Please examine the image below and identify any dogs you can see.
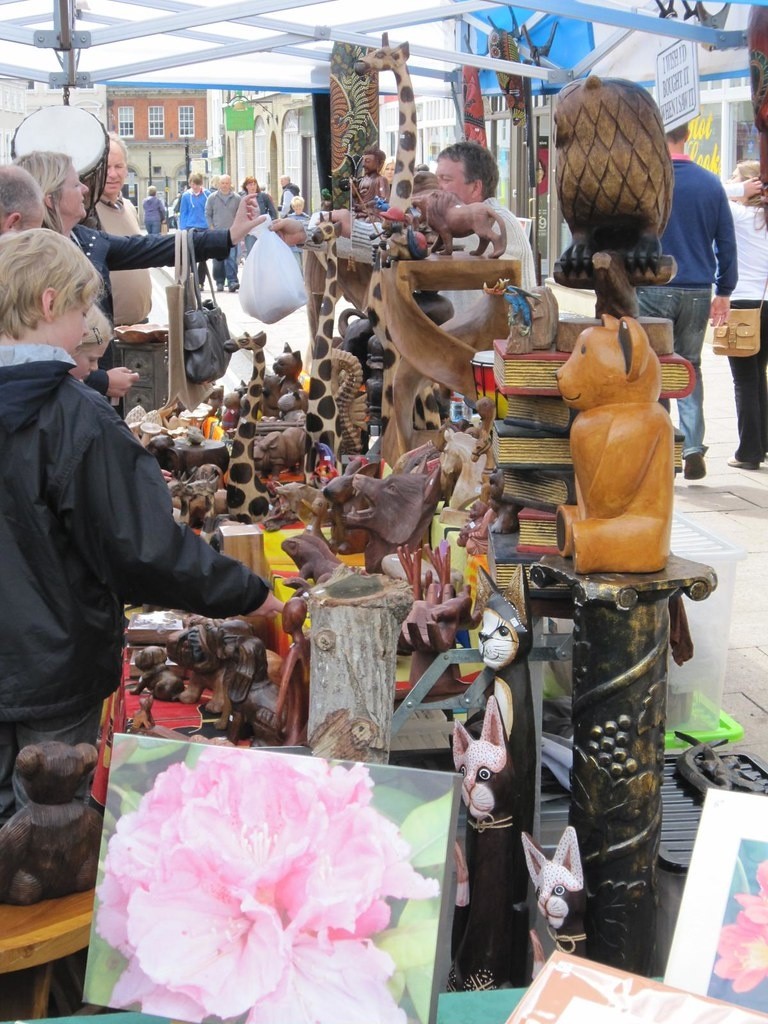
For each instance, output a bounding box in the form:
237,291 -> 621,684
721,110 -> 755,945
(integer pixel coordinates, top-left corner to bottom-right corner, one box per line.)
272,342 -> 303,395
128,645 -> 185,702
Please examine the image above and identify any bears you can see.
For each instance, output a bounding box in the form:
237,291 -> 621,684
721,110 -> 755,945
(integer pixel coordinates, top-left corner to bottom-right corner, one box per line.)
554,312 -> 676,574
0,738 -> 104,906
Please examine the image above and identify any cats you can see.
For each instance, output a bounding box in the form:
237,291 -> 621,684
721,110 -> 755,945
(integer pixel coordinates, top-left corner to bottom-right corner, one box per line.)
451,561 -> 594,988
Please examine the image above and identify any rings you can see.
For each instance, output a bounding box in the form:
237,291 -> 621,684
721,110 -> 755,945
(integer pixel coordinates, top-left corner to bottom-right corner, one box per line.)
755,182 -> 758,185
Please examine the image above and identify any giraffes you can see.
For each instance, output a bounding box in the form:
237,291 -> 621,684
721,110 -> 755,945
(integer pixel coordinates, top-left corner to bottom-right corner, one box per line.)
350,30 -> 442,471
297,211 -> 344,491
222,330 -> 272,523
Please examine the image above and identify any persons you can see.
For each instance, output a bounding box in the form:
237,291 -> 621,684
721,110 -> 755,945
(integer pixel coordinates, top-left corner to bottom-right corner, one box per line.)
355,148 -> 391,217
0,130 -> 311,827
638,119 -> 768,480
268,142 -> 537,336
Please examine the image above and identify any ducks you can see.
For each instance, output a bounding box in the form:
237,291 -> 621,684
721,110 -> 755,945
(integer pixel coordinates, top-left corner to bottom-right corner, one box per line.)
301,496 -> 332,552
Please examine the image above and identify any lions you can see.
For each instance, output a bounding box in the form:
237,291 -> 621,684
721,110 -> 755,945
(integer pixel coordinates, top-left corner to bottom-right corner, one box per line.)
411,191 -> 508,259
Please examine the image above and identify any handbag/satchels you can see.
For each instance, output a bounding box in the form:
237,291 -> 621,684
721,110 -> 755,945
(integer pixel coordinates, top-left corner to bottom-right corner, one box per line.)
239,214 -> 309,324
162,228 -> 232,414
712,307 -> 761,357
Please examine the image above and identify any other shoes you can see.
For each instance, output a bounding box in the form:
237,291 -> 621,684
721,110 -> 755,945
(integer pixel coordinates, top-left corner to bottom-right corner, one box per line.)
217,283 -> 224,291
728,459 -> 760,469
199,283 -> 204,291
684,453 -> 706,480
228,281 -> 240,292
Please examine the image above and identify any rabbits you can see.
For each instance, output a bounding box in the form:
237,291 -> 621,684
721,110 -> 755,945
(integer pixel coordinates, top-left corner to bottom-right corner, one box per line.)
322,459 -> 378,556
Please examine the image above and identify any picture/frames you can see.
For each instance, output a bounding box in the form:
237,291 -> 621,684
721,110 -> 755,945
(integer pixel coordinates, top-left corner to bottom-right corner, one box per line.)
663,785 -> 768,1014
